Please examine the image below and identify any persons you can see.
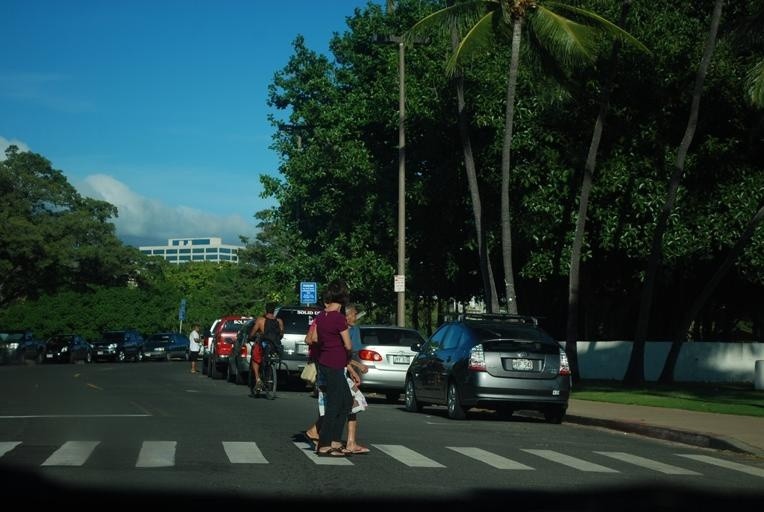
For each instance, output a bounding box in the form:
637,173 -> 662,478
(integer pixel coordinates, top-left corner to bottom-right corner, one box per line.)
346,304 -> 369,378
250,303 -> 284,390
311,280 -> 353,456
189,324 -> 205,374
305,291 -> 371,453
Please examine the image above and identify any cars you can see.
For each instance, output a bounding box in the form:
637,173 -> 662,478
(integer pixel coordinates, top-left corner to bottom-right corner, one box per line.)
225,319 -> 254,384
350,325 -> 426,401
141,333 -> 190,362
36,334 -> 94,364
203,315 -> 257,379
404,310 -> 571,424
0,330 -> 36,365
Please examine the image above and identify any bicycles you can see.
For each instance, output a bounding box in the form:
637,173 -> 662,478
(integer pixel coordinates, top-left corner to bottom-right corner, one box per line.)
248,337 -> 277,400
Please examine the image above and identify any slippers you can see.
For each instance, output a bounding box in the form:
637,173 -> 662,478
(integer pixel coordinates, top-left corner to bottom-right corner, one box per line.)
336,445 -> 353,456
346,444 -> 370,454
316,448 -> 346,458
304,432 -> 319,449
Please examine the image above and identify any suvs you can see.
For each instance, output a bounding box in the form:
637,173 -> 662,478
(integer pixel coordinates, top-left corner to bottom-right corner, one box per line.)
275,307 -> 329,388
89,331 -> 143,363
